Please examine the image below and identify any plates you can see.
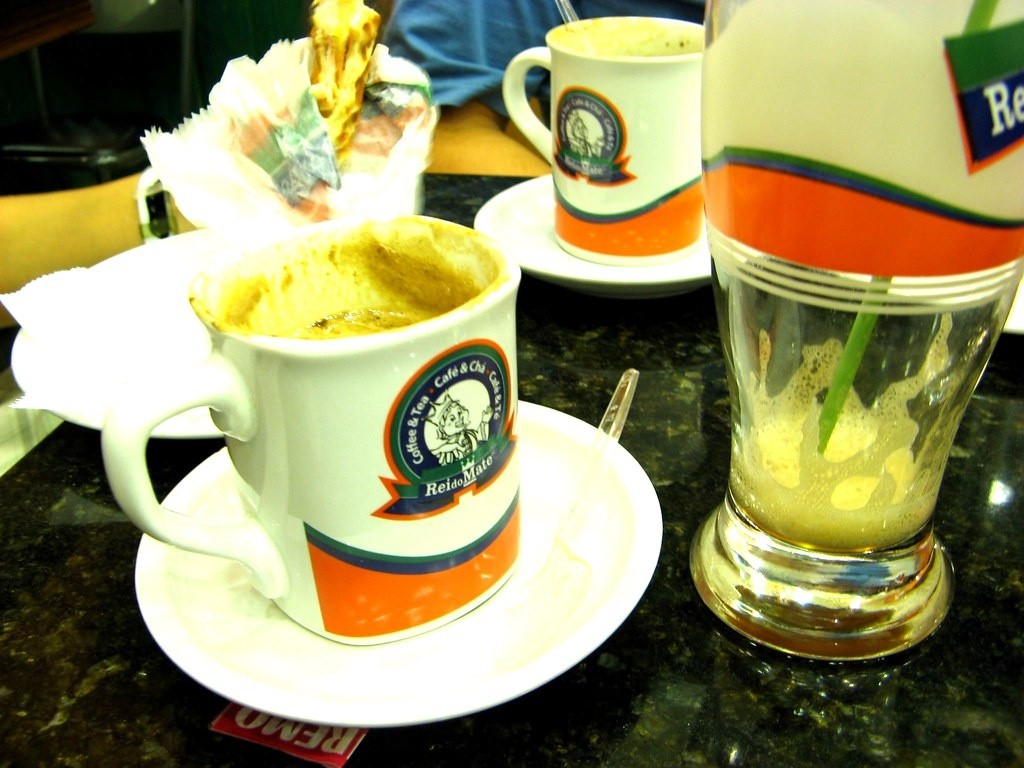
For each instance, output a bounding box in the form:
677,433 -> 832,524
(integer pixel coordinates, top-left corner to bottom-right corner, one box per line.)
133,398 -> 665,728
10,228 -> 297,440
474,174 -> 712,299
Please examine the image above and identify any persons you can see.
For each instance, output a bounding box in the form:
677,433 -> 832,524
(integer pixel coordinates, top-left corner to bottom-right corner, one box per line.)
0,162 -> 200,329
382,0 -> 706,177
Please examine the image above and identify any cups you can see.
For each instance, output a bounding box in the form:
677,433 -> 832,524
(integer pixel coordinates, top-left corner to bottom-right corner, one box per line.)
501,17 -> 705,266
101,214 -> 523,646
688,0 -> 1024,659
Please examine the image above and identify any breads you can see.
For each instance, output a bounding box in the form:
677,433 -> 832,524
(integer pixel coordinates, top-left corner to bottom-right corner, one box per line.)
307,0 -> 382,169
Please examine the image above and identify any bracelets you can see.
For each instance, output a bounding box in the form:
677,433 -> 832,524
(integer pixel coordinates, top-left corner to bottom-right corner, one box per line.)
136,165 -> 179,243
